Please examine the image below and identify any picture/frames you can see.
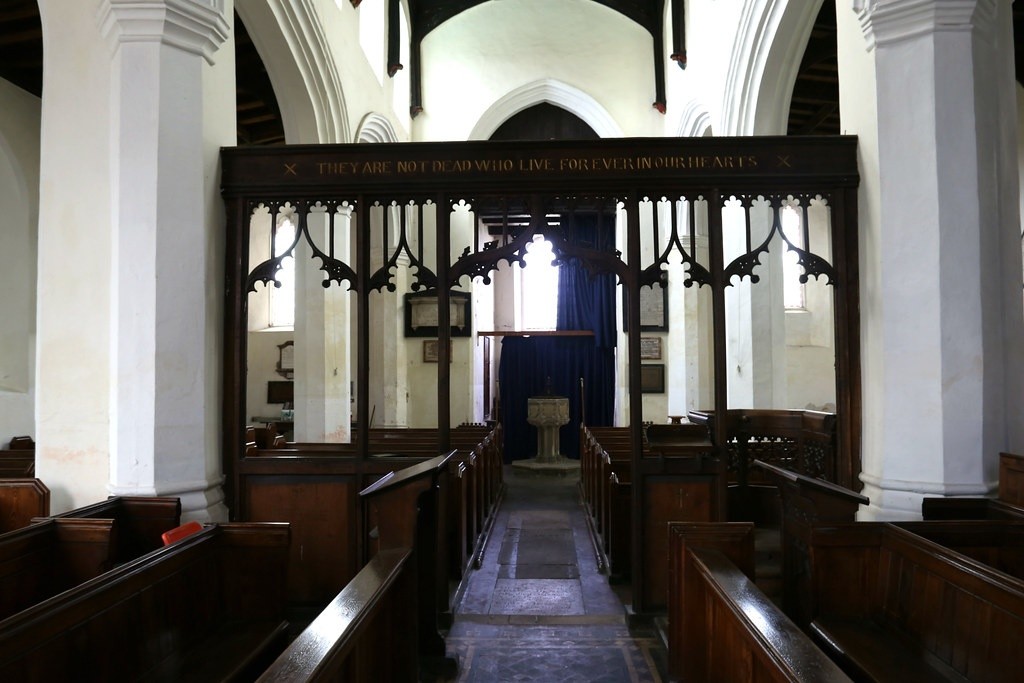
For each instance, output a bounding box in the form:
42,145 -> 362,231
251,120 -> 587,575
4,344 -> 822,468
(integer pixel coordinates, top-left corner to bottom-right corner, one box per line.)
642,364 -> 665,393
641,337 -> 661,360
423,340 -> 453,363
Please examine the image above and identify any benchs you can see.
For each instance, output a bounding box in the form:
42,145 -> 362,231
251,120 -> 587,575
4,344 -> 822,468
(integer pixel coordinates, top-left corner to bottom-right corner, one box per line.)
0,420 -> 504,683
579,409 -> 1024,683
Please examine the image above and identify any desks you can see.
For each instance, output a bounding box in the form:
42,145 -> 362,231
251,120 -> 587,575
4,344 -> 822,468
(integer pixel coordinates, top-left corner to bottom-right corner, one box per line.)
512,398 -> 581,470
